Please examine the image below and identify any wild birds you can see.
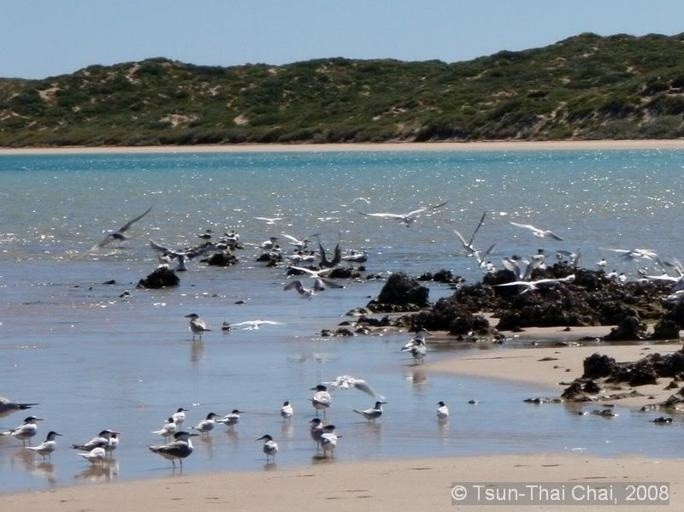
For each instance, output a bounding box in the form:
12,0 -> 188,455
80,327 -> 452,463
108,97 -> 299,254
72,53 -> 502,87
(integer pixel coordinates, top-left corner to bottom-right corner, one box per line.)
448,212 -> 684,296
148,407 -> 247,471
359,202 -> 448,229
184,313 -> 212,340
221,319 -> 282,330
308,375 -> 388,460
401,328 -> 433,363
281,399 -> 294,423
257,433 -> 278,462
149,217 -> 368,299
437,401 -> 449,421
352,197 -> 370,204
71,429 -> 121,465
77,207 -> 151,260
0,398 -> 64,461
